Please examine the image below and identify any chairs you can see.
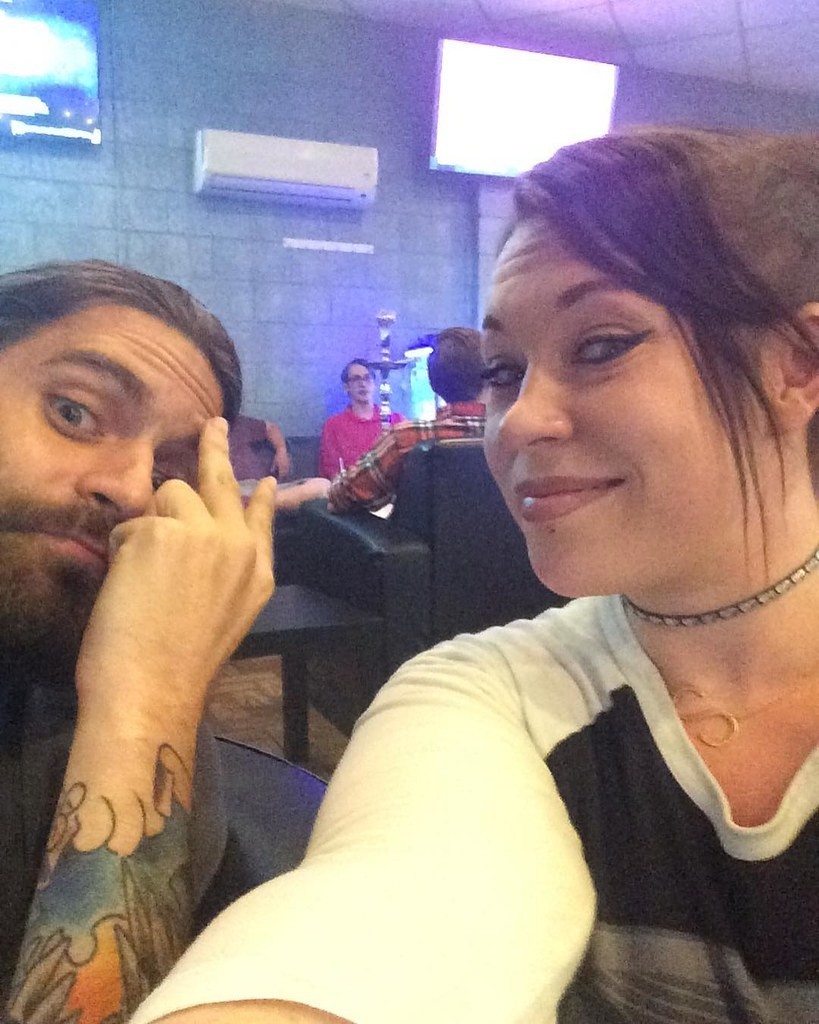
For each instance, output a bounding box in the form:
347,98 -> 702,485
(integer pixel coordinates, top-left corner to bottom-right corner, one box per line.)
190,735 -> 327,937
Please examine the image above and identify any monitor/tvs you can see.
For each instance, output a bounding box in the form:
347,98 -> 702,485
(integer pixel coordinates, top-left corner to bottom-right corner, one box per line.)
424,37 -> 621,182
0,0 -> 102,145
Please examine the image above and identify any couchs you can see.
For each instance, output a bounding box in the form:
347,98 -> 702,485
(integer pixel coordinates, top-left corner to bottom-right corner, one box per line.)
287,437 -> 576,741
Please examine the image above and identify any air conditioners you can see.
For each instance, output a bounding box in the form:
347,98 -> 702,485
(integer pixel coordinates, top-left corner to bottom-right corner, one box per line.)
191,128 -> 379,211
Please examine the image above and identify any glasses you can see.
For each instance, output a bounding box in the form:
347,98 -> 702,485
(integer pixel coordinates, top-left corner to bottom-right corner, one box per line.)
346,374 -> 373,382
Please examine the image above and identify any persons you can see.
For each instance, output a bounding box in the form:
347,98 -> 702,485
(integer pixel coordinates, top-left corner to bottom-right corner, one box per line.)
127,125 -> 819,1024
0,261 -> 279,1021
228,325 -> 494,586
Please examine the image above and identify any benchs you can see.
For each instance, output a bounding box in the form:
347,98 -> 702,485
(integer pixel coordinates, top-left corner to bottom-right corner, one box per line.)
273,435 -> 321,583
230,585 -> 372,766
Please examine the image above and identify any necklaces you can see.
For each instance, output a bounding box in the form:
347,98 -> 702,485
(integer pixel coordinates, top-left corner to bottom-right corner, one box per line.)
621,544 -> 819,629
666,684 -> 802,748
